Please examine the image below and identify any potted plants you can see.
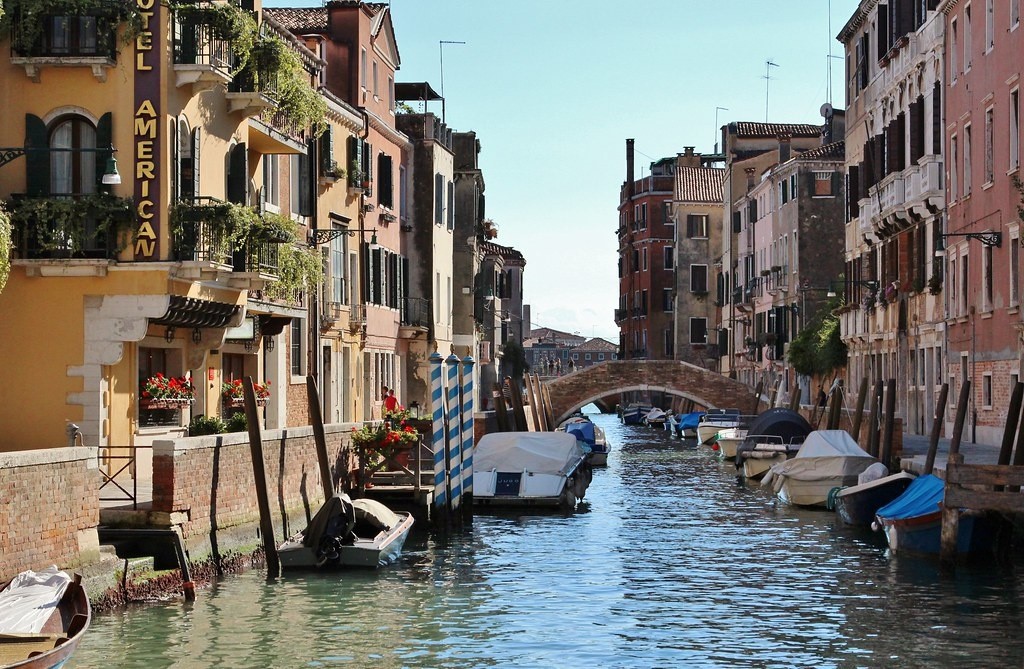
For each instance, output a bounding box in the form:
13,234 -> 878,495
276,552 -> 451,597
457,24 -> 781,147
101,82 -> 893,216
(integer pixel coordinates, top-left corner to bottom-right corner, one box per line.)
758,332 -> 777,347
771,266 -> 781,272
1,188 -> 301,269
165,0 -> 332,142
831,309 -> 843,316
928,275 -> 943,297
324,159 -> 370,188
417,414 -> 433,432
840,306 -> 851,313
848,301 -> 860,310
473,217 -> 499,241
760,270 -> 770,276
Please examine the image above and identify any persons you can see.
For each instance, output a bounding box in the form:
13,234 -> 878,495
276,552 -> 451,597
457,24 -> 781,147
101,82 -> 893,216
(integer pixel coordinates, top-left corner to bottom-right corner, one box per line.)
567,357 -> 575,374
383,386 -> 389,400
384,389 -> 399,413
818,385 -> 827,406
538,357 -> 561,376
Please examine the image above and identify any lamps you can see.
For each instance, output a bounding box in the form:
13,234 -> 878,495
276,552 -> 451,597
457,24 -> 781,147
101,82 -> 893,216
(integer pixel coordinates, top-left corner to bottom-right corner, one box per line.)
410,401 -> 420,420
484,309 -> 512,322
703,319 -> 751,338
0,142 -> 122,185
934,230 -> 1003,257
487,326 -> 514,337
769,304 -> 800,318
309,227 -> 379,250
472,284 -> 494,300
165,324 -> 177,343
826,280 -> 880,298
192,327 -> 202,346
244,341 -> 252,352
267,335 -> 274,353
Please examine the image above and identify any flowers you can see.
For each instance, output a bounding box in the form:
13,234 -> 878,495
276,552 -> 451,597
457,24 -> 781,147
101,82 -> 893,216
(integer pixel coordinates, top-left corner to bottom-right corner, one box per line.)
221,376 -> 272,400
347,404 -> 419,489
885,286 -> 898,305
137,372 -> 198,400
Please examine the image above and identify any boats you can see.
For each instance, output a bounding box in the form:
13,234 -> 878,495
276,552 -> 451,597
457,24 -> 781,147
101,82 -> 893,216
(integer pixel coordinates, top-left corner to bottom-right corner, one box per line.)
829,470 -> 917,526
278,498 -> 415,570
470,432 -> 594,507
760,430 -> 881,507
874,475 -> 974,556
554,413 -> 611,467
736,407 -> 813,480
0,565 -> 92,669
623,403 -> 707,438
696,408 -> 749,459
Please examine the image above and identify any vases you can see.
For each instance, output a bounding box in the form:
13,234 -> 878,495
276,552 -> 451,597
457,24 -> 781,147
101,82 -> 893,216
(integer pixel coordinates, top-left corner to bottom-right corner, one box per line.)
225,397 -> 271,407
877,58 -> 890,69
156,400 -> 167,408
166,400 -> 178,409
139,399 -> 157,409
386,441 -> 413,471
352,469 -> 373,489
888,47 -> 900,59
176,400 -> 188,410
895,37 -> 909,48
